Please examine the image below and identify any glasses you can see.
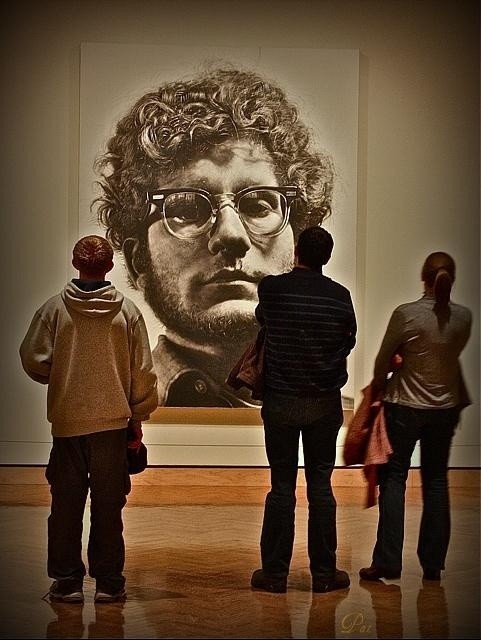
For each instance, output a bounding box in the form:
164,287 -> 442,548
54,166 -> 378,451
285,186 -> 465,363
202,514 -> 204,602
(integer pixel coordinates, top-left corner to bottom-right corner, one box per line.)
141,183 -> 297,240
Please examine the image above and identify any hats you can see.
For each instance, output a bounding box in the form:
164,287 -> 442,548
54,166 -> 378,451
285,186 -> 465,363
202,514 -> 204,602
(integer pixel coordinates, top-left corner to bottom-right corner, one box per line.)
127,435 -> 146,474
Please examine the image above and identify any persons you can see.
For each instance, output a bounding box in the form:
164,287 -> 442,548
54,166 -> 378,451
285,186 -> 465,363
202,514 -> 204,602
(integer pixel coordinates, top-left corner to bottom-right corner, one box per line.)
357,248 -> 471,584
43,602 -> 129,640
20,236 -> 158,603
250,226 -> 352,593
87,60 -> 354,409
254,588 -> 352,639
358,578 -> 453,640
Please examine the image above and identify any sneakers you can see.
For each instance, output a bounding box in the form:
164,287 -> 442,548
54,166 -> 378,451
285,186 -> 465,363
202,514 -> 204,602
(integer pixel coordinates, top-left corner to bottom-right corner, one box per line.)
250,569 -> 289,594
93,578 -> 127,603
311,570 -> 349,593
42,579 -> 84,604
359,565 -> 401,580
422,569 -> 441,581
